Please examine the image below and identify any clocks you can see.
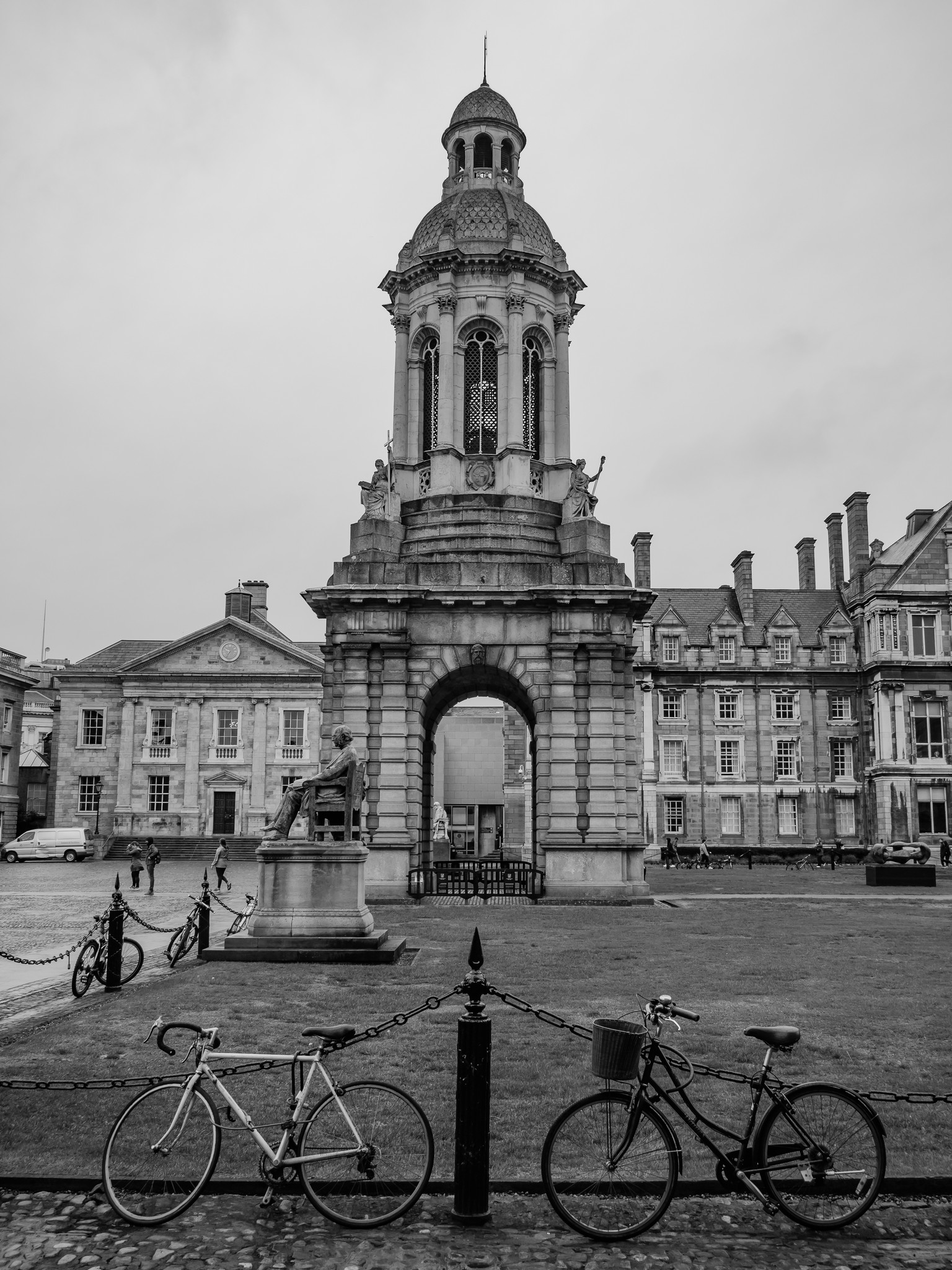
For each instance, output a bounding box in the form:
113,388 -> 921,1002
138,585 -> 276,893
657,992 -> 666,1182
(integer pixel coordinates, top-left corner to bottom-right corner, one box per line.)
219,641 -> 241,662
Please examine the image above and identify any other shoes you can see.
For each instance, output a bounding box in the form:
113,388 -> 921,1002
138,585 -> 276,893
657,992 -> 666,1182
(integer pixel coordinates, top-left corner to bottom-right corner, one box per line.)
834,861 -> 837,865
944,866 -> 948,869
697,866 -> 703,869
144,891 -> 154,895
709,866 -> 713,869
129,885 -> 140,890
227,882 -> 231,890
823,863 -> 826,866
663,864 -> 670,867
839,864 -> 844,867
816,866 -> 821,867
212,887 -> 220,892
948,863 -> 952,867
939,866 -> 945,868
670,865 -> 675,867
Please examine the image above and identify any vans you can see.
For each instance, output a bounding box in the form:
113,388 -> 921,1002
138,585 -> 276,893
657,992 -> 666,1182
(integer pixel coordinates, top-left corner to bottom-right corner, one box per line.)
1,826 -> 95,863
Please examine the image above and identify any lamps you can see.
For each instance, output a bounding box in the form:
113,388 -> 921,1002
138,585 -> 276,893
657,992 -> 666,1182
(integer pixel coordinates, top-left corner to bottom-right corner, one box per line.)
934,751 -> 939,757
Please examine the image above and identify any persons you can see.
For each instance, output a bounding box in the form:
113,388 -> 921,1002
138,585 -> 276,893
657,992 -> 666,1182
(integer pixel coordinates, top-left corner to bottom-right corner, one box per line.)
260,725 -> 357,841
432,802 -> 447,833
663,837 -> 679,867
697,839 -> 713,869
126,841 -> 145,890
567,459 -> 603,518
939,838 -> 952,869
357,446 -> 396,521
144,836 -> 158,895
497,824 -> 503,849
211,838 -> 232,892
834,839 -> 845,867
815,838 -> 826,868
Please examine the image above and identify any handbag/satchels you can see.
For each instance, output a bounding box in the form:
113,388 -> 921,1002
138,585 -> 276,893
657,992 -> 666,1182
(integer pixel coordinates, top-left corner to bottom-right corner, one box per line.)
815,848 -> 821,853
150,844 -> 161,864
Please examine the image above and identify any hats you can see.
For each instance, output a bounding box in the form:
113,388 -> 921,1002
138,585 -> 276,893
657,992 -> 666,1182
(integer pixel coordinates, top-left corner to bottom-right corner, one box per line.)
818,838 -> 822,841
837,839 -> 842,843
145,836 -> 153,846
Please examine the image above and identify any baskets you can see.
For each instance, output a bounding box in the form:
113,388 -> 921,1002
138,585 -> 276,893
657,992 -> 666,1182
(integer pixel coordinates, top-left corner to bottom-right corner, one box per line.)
591,1019 -> 649,1081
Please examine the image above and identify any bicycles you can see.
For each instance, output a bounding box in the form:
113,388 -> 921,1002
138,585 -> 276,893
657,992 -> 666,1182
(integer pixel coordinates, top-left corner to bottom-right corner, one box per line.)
707,854 -> 734,870
101,1014 -> 434,1230
225,892 -> 258,937
675,853 -> 708,870
786,854 -> 814,871
162,894 -> 215,969
539,992 -> 888,1244
70,915 -> 144,998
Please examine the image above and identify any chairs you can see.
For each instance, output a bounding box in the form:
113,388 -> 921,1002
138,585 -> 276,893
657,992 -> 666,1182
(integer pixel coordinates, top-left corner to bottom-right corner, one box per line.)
306,761 -> 367,841
